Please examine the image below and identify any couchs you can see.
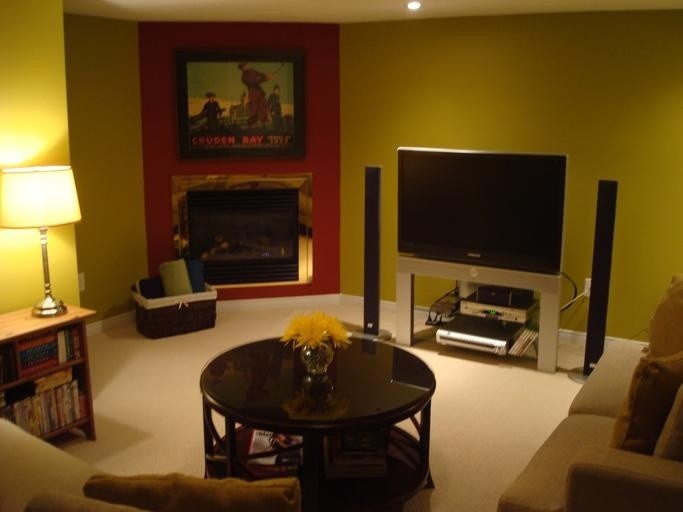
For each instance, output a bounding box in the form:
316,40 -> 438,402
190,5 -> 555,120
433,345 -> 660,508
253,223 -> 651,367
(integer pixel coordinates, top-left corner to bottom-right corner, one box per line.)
0,417 -> 151,511
497,338 -> 683,512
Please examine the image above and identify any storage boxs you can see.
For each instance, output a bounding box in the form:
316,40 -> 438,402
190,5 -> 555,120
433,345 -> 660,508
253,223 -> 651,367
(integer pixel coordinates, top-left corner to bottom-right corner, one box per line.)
133,282 -> 218,339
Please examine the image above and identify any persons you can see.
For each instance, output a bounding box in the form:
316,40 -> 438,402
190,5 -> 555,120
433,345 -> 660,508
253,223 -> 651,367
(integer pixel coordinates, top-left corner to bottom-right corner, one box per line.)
236,61 -> 273,126
266,83 -> 284,129
195,91 -> 224,130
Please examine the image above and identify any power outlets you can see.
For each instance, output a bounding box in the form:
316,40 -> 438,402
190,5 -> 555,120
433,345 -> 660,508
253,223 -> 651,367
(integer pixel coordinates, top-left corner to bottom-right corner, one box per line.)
584,279 -> 591,297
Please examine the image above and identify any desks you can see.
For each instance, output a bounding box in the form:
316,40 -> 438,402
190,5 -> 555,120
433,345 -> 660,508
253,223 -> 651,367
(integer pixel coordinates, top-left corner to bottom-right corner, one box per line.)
395,256 -> 561,373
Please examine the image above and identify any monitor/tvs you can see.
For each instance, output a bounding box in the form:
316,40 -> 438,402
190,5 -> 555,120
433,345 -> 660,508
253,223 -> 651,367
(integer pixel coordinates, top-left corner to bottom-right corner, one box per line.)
397,147 -> 568,274
176,50 -> 306,160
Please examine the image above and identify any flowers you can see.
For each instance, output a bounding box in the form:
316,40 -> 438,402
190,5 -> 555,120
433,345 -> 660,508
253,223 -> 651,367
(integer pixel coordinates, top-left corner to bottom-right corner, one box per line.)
281,391 -> 347,420
280,312 -> 352,349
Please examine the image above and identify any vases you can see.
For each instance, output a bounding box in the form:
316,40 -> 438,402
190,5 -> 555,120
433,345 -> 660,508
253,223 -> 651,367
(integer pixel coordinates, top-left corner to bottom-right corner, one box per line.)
301,342 -> 334,375
303,375 -> 334,397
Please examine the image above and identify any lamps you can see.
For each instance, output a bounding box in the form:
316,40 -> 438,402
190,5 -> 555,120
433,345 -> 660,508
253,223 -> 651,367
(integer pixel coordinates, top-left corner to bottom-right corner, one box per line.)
0,166 -> 82,318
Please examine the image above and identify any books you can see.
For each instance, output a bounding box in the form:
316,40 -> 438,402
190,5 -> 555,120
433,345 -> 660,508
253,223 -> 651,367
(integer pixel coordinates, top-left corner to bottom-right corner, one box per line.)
247,428 -> 306,471
0,325 -> 89,440
337,429 -> 376,456
506,317 -> 541,358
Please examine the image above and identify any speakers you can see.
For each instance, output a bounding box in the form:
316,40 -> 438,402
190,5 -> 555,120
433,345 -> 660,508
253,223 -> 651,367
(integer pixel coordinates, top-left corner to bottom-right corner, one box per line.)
354,166 -> 392,341
568,180 -> 618,385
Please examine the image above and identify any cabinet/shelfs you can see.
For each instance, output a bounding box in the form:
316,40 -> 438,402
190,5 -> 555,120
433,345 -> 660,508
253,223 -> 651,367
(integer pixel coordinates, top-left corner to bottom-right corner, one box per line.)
0,305 -> 98,441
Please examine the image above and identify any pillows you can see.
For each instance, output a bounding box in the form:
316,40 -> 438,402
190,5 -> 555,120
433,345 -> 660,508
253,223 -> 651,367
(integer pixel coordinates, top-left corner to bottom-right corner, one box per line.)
609,349 -> 683,455
645,273 -> 683,357
82,472 -> 302,512
